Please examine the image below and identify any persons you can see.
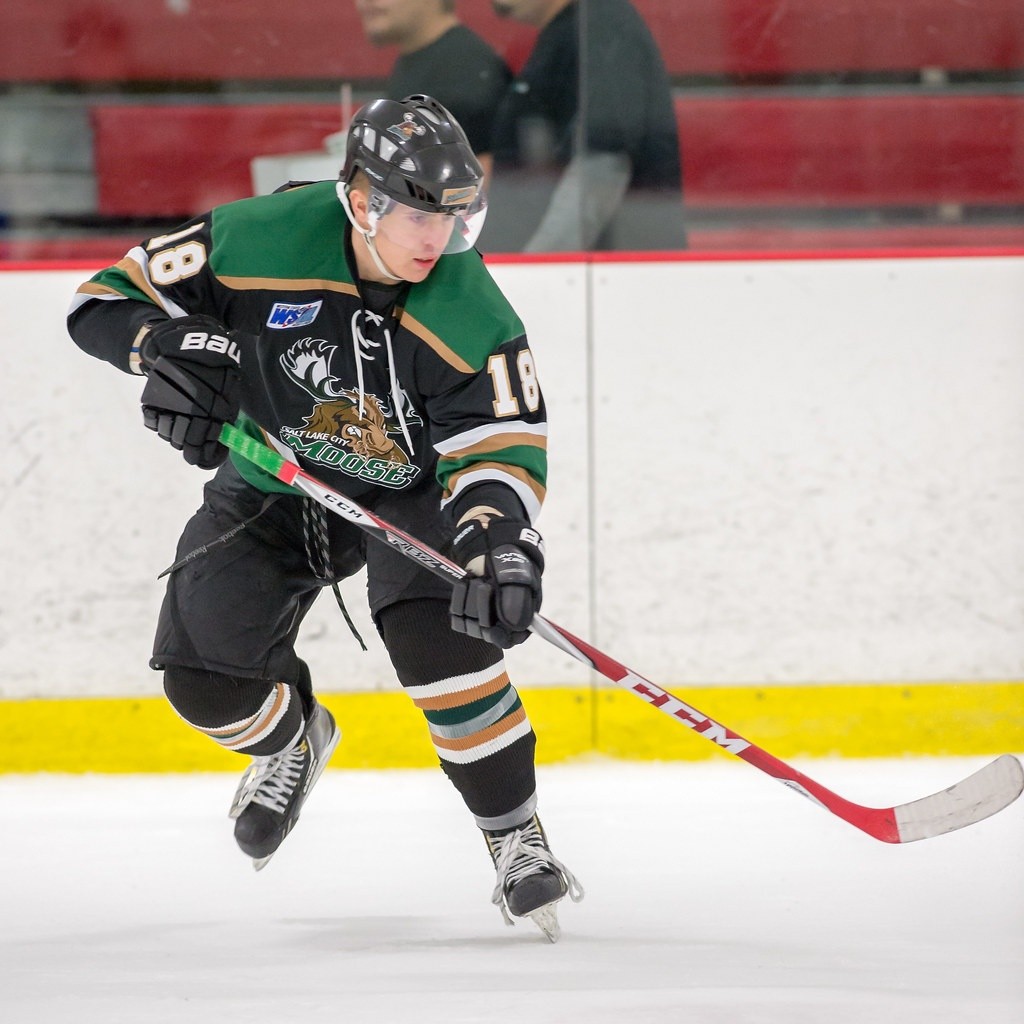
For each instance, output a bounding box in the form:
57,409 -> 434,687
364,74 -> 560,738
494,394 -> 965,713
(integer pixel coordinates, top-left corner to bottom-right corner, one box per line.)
66,94 -> 586,943
466,0 -> 688,256
356,0 -> 515,192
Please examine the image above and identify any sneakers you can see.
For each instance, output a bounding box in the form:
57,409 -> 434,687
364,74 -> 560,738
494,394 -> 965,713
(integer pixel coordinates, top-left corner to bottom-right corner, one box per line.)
228,657 -> 340,872
481,810 -> 585,943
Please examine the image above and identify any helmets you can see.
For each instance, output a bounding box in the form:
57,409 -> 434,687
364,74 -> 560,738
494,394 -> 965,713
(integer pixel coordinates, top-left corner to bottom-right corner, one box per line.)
344,94 -> 485,213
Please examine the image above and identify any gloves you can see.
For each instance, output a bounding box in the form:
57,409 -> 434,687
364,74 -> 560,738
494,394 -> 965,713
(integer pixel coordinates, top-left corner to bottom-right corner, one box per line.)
141,314 -> 243,470
439,518 -> 546,649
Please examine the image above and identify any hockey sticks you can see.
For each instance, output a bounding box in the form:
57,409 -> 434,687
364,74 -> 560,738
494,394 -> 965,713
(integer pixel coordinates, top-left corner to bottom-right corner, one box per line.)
223,405 -> 1024,845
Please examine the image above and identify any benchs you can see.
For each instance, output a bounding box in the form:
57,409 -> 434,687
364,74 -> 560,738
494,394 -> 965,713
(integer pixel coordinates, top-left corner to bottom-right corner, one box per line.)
0,0 -> 1024,246
